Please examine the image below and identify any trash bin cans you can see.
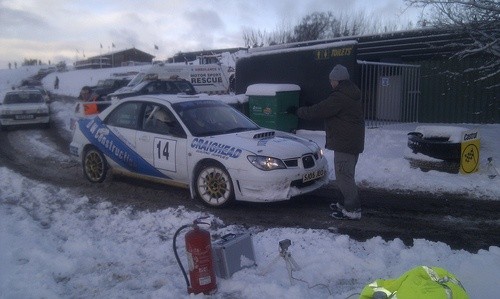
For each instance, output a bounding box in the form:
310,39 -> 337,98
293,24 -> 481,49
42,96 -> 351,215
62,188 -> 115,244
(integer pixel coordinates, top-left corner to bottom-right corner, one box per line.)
244,85 -> 300,135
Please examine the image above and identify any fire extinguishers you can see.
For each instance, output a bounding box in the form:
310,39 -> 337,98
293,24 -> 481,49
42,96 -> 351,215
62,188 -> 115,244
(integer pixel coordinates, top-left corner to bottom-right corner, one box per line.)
172,215 -> 218,295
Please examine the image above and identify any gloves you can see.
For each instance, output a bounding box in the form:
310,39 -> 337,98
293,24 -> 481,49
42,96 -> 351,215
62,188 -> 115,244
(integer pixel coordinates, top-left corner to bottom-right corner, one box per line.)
286,105 -> 297,116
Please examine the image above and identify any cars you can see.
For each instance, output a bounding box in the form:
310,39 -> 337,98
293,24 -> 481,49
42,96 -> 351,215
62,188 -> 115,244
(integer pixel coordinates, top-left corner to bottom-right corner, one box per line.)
0,90 -> 50,128
69,94 -> 331,209
99,76 -> 197,113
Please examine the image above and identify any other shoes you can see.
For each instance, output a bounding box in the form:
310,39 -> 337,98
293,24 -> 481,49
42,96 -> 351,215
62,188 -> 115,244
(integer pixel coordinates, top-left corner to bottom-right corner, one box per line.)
331,211 -> 351,219
329,203 -> 342,212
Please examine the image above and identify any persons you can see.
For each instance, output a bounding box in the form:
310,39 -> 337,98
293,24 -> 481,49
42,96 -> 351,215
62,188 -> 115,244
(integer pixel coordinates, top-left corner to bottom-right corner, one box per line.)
80,86 -> 109,115
287,65 -> 366,220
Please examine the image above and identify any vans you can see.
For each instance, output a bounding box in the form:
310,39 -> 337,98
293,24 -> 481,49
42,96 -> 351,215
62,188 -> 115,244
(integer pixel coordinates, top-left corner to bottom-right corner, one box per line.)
127,63 -> 231,96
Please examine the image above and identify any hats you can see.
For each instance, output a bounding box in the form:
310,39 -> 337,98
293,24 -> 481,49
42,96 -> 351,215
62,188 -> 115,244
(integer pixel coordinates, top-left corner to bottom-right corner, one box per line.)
328,64 -> 350,80
